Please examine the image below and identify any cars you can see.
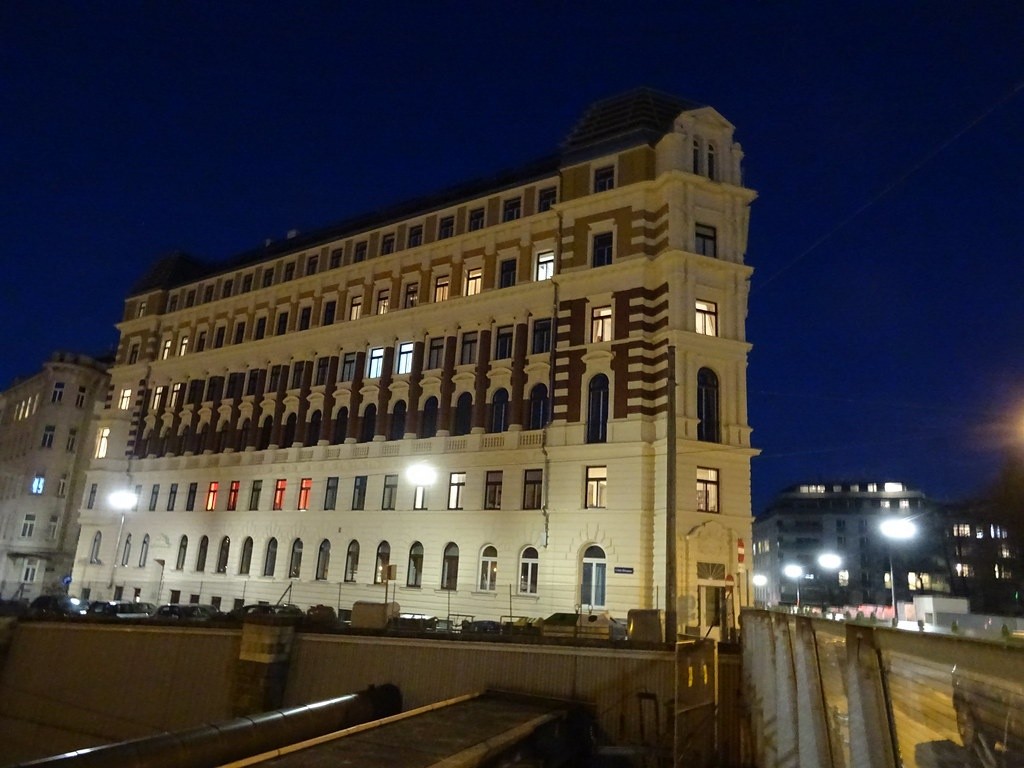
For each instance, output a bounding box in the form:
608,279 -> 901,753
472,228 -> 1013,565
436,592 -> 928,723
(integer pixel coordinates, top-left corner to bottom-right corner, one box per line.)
26,594 -> 90,617
225,604 -> 303,615
85,600 -> 158,618
153,604 -> 219,621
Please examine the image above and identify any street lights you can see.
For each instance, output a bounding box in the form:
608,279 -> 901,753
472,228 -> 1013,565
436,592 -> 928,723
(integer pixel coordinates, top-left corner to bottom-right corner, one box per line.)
789,568 -> 799,605
885,523 -> 904,617
